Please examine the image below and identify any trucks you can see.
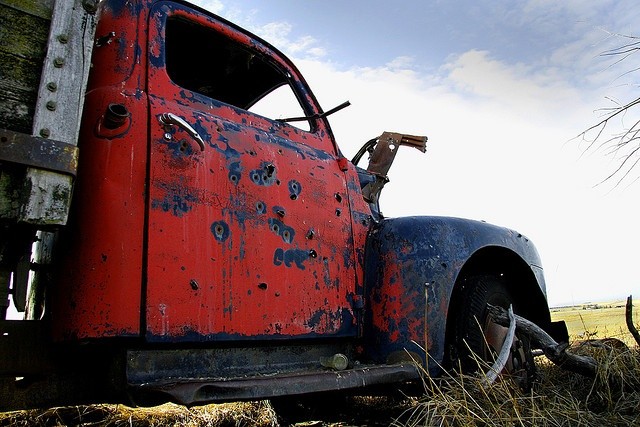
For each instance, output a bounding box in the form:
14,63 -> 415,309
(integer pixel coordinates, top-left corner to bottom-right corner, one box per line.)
2,0 -> 573,424
